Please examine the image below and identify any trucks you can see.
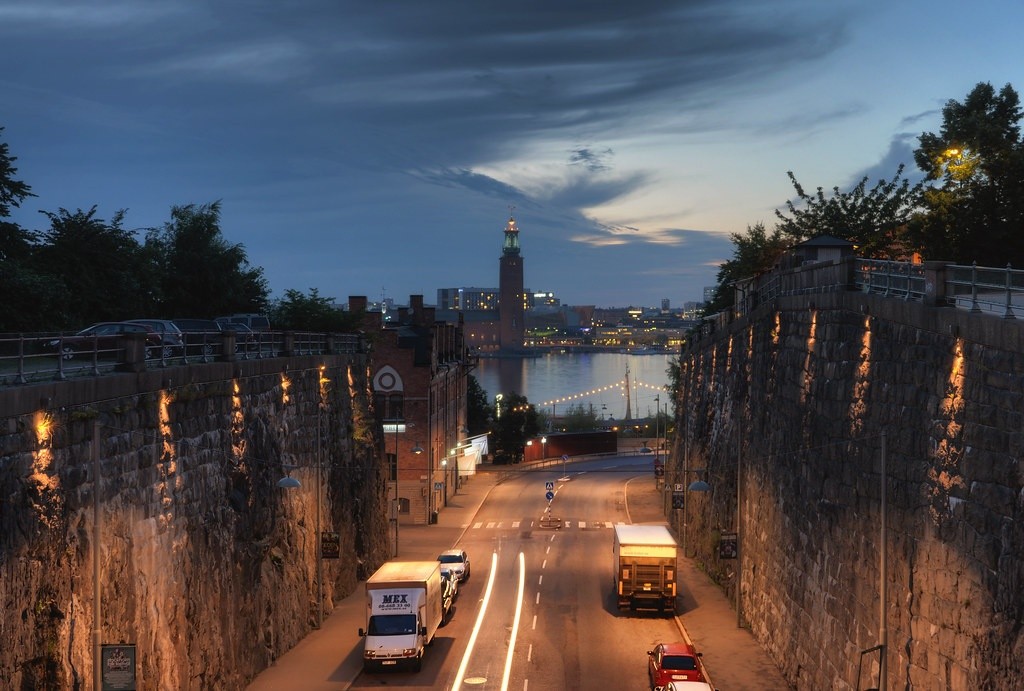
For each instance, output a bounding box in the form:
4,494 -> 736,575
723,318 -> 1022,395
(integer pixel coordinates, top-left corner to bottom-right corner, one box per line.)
611,522 -> 679,617
356,561 -> 444,672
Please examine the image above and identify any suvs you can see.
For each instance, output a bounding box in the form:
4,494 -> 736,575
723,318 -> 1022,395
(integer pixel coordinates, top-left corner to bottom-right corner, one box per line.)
436,549 -> 471,583
100,318 -> 184,358
173,313 -> 272,356
41,322 -> 162,361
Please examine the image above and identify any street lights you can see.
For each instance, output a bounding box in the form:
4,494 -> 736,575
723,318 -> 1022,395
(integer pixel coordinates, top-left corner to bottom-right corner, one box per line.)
541,436 -> 547,469
653,394 -> 659,463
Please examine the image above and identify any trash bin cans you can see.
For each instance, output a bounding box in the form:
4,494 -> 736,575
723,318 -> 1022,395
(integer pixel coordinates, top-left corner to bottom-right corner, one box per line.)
431,510 -> 440,524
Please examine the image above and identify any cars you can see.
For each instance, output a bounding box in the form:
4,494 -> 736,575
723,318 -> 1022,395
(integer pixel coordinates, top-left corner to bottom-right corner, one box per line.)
440,567 -> 459,596
647,642 -> 704,691
440,575 -> 454,627
662,682 -> 720,691
